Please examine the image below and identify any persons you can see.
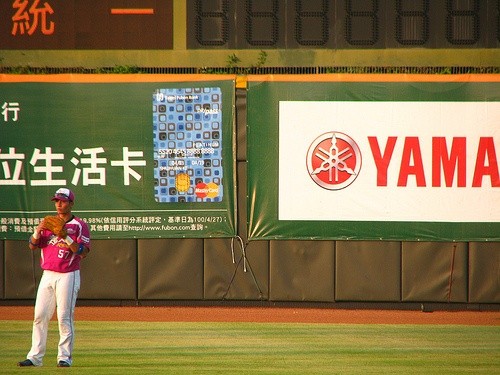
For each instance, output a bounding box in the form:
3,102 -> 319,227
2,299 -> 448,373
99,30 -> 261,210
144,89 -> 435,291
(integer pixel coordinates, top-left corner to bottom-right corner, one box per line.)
16,187 -> 91,368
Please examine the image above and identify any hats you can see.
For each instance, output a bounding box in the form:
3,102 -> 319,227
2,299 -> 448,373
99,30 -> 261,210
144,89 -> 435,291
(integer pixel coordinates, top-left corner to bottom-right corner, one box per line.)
51,188 -> 74,201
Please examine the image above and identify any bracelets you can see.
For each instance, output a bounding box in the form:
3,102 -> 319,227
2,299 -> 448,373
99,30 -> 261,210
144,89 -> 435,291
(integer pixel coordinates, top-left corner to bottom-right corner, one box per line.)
73,244 -> 84,254
29,237 -> 39,247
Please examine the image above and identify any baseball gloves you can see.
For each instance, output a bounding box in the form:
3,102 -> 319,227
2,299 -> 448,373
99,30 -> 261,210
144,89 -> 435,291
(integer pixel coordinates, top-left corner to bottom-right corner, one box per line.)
43,215 -> 67,239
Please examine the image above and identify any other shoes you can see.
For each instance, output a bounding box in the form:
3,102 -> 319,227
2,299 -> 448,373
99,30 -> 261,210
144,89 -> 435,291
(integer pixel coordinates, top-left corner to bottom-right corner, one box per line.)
59,360 -> 70,367
20,359 -> 33,366
